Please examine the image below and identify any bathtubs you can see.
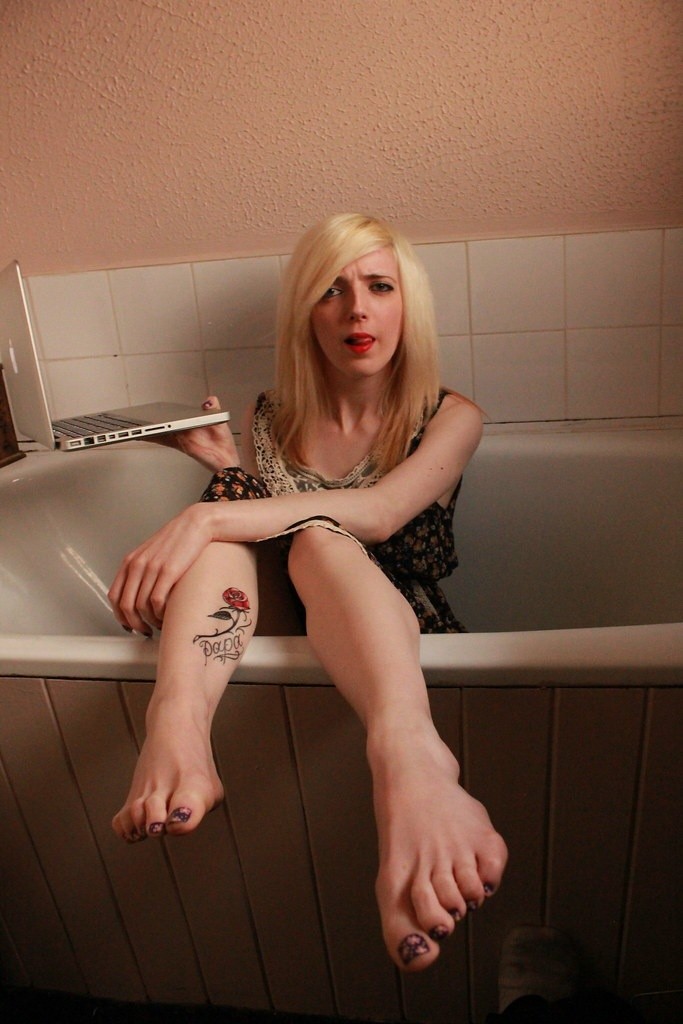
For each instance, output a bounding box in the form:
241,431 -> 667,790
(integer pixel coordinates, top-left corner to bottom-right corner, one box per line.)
0,419 -> 683,681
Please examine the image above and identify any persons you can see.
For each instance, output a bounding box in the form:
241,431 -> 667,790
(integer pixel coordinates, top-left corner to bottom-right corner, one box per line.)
105,208 -> 510,973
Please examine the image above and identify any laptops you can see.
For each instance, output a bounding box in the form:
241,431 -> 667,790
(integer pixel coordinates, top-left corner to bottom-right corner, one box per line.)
0,260 -> 231,452
